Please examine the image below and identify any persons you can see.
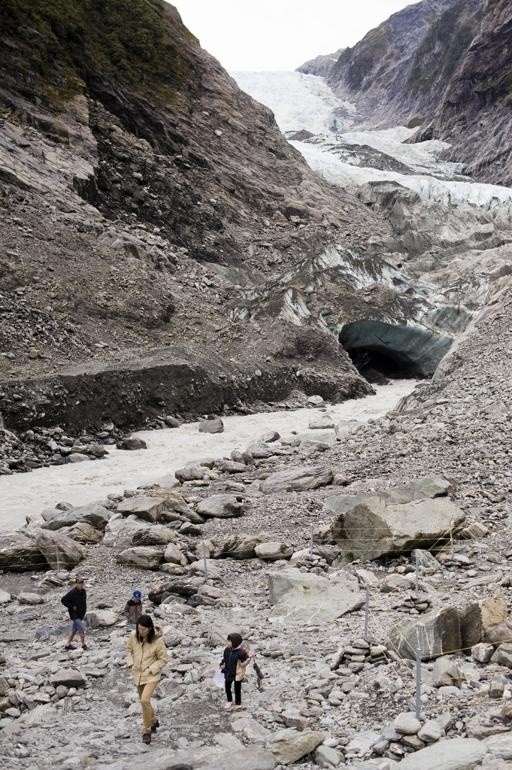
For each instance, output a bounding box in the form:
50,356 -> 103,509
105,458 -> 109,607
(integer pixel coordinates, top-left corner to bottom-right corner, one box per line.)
219,633 -> 251,710
60,575 -> 88,651
126,615 -> 167,743
123,590 -> 142,630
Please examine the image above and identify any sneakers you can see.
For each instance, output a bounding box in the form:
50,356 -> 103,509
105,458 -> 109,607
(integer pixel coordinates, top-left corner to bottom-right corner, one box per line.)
151,719 -> 159,732
224,702 -> 242,710
65,644 -> 76,649
82,644 -> 87,651
143,733 -> 151,743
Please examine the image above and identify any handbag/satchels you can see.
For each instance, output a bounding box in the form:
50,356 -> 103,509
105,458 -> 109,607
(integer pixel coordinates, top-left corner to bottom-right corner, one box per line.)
235,661 -> 248,682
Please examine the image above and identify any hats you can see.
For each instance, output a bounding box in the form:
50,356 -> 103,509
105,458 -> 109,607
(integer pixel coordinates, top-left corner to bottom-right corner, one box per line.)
134,590 -> 141,599
229,632 -> 242,648
76,578 -> 84,583
